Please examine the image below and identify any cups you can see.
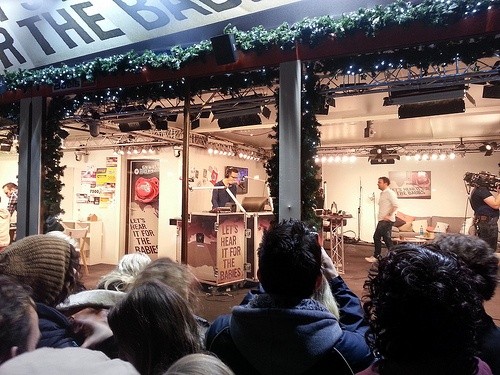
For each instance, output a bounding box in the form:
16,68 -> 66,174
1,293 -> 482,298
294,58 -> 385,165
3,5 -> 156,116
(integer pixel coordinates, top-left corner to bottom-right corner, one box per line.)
429,233 -> 435,238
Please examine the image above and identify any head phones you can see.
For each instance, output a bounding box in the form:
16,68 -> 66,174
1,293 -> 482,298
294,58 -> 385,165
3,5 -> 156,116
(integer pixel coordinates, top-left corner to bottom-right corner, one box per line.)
224,167 -> 234,178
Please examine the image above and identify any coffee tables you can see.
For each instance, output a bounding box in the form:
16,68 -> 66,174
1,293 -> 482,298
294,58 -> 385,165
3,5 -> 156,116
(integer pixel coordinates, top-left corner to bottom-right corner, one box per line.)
391,237 -> 427,245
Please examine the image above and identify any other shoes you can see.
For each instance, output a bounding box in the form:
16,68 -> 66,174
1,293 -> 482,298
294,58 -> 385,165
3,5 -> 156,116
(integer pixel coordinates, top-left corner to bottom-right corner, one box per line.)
365,255 -> 378,262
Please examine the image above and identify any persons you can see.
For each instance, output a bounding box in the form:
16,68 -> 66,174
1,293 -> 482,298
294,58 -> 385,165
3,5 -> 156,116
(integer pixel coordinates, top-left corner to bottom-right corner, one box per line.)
354,233 -> 500,375
0,183 -> 18,247
205,219 -> 376,375
0,231 -> 235,375
211,168 -> 238,208
470,171 -> 500,253
365,177 -> 398,262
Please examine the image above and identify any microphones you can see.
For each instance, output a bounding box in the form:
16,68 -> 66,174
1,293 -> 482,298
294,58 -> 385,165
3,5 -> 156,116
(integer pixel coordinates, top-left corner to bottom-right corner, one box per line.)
235,181 -> 245,189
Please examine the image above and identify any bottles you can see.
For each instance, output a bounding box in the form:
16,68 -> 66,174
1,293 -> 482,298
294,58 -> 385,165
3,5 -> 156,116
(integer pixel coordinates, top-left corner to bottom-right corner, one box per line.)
420,225 -> 424,237
436,226 -> 440,238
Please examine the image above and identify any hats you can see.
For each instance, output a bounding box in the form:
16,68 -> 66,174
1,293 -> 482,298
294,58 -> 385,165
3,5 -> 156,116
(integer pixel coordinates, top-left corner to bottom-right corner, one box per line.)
46,231 -> 78,248
0,234 -> 71,306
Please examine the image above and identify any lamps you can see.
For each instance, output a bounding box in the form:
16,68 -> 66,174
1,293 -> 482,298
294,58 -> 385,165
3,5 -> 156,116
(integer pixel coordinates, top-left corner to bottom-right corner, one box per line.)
112,144 -> 157,156
314,152 -> 357,163
405,148 -> 456,162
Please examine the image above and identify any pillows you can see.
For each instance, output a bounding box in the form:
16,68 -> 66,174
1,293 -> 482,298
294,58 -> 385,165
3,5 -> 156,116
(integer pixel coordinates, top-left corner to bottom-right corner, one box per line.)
433,222 -> 449,234
460,218 -> 474,235
411,219 -> 427,232
396,211 -> 416,232
392,216 -> 406,227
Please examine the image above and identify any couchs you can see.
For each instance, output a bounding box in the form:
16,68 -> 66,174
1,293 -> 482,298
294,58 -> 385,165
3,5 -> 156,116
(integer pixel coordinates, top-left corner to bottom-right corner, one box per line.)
391,215 -> 475,239
